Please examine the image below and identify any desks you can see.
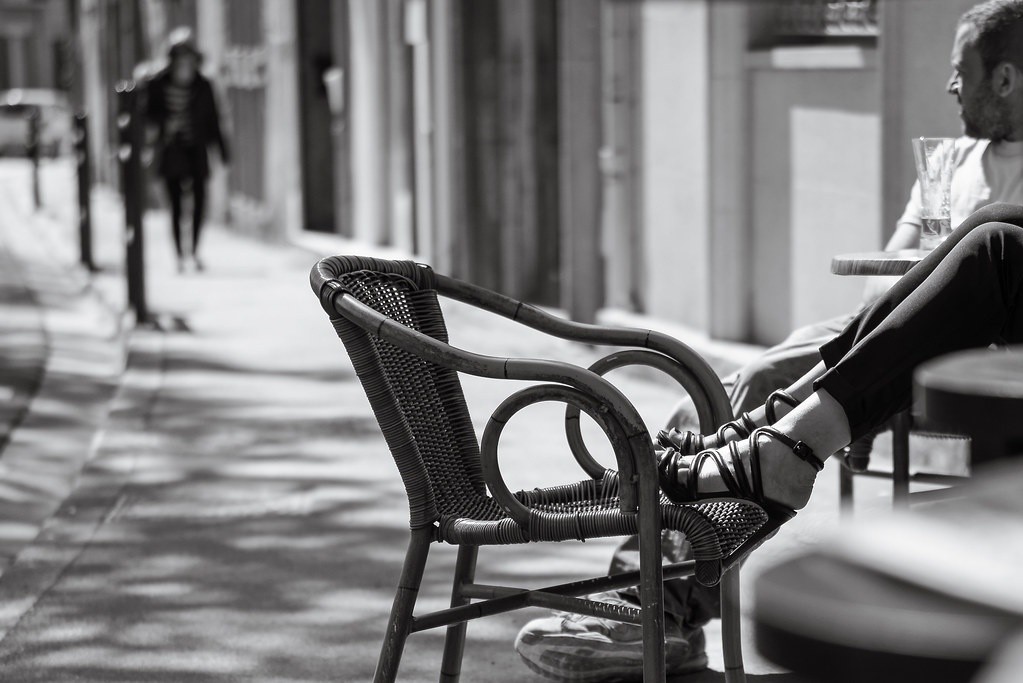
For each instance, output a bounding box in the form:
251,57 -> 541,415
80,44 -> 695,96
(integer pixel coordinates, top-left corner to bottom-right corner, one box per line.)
833,251 -> 968,509
918,347 -> 1023,475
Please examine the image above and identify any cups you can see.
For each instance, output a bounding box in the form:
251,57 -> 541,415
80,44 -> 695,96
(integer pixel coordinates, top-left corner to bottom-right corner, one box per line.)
912,137 -> 957,252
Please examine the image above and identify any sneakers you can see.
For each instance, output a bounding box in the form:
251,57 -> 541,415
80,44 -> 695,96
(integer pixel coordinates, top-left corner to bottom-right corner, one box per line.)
514,589 -> 709,682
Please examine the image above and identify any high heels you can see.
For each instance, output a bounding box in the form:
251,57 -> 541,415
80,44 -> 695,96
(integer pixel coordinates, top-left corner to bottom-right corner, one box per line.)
656,388 -> 801,454
653,426 -> 822,568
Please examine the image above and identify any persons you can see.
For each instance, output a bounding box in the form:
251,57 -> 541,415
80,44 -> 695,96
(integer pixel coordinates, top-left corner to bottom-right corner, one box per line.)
135,24 -> 232,277
648,202 -> 1022,568
514,0 -> 1023,682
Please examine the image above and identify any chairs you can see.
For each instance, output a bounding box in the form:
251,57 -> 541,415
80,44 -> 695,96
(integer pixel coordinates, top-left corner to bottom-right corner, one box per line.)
309,254 -> 779,683
